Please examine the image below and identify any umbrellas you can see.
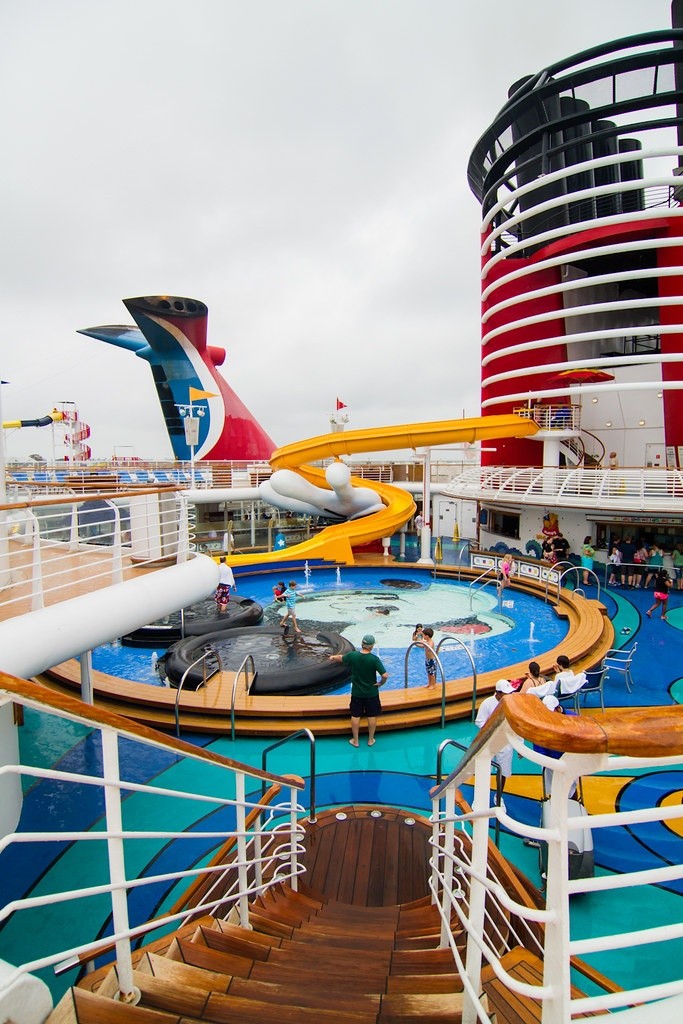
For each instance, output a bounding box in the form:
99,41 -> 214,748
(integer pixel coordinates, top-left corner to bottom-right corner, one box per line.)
546,368 -> 614,430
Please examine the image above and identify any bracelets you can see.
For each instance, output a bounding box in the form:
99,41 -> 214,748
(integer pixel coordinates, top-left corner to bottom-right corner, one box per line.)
416,635 -> 418,637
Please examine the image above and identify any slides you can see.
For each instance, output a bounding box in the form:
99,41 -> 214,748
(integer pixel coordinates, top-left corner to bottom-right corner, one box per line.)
213,414 -> 538,569
64,411 -> 91,461
3,412 -> 61,429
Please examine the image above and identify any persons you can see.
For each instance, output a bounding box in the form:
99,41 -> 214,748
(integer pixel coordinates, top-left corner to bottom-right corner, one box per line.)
646,570 -> 673,620
671,543 -> 683,591
533,696 -> 577,800
411,623 -> 437,689
272,581 -> 305,632
542,533 -> 570,565
223,531 -> 235,554
214,556 -> 236,611
330,634 -> 388,747
414,510 -> 422,546
581,536 -> 595,585
476,680 -> 525,813
553,655 -> 574,682
519,661 -> 546,693
607,536 -> 664,590
498,554 -> 513,596
520,398 -> 571,427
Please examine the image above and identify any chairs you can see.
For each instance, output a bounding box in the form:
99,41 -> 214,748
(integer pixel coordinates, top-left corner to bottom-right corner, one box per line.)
601,641 -> 639,693
541,670 -> 588,714
576,666 -> 610,717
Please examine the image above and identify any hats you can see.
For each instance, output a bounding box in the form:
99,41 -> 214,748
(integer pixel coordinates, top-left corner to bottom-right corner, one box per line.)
543,695 -> 560,712
495,679 -> 516,694
362,634 -> 376,644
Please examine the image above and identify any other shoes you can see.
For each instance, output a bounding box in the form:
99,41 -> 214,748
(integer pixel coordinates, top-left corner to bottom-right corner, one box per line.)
645,611 -> 652,618
614,583 -> 616,586
631,585 -> 635,589
621,583 -> 625,589
661,616 -> 667,619
643,585 -> 647,588
493,796 -> 507,814
583,582 -> 592,586
635,584 -> 641,588
628,584 -> 632,588
607,582 -> 614,585
